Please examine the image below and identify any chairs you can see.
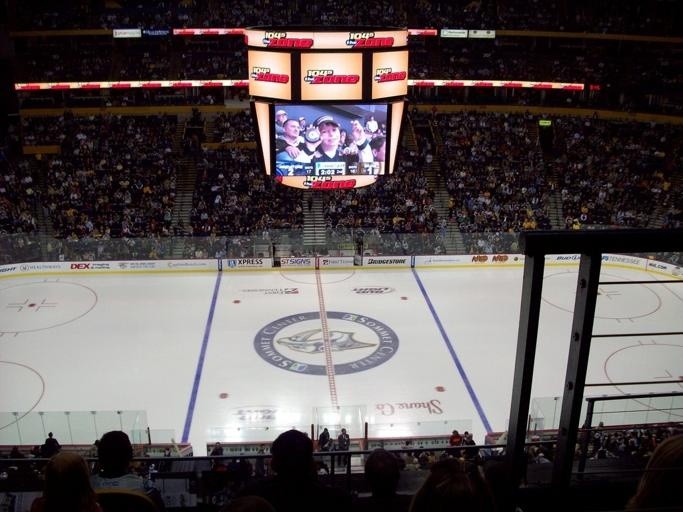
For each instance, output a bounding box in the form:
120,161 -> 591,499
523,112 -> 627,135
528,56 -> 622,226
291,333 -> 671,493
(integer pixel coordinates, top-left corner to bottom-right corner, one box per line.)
95,485 -> 160,512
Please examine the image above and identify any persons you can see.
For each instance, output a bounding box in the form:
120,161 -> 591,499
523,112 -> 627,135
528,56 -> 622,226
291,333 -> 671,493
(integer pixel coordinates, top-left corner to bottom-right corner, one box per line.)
0,88 -> 681,266
0,428 -> 682,512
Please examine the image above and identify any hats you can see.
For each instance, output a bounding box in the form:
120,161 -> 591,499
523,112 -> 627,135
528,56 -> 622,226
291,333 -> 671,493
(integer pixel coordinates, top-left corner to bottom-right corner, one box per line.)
312,115 -> 339,128
298,117 -> 305,122
275,109 -> 288,116
